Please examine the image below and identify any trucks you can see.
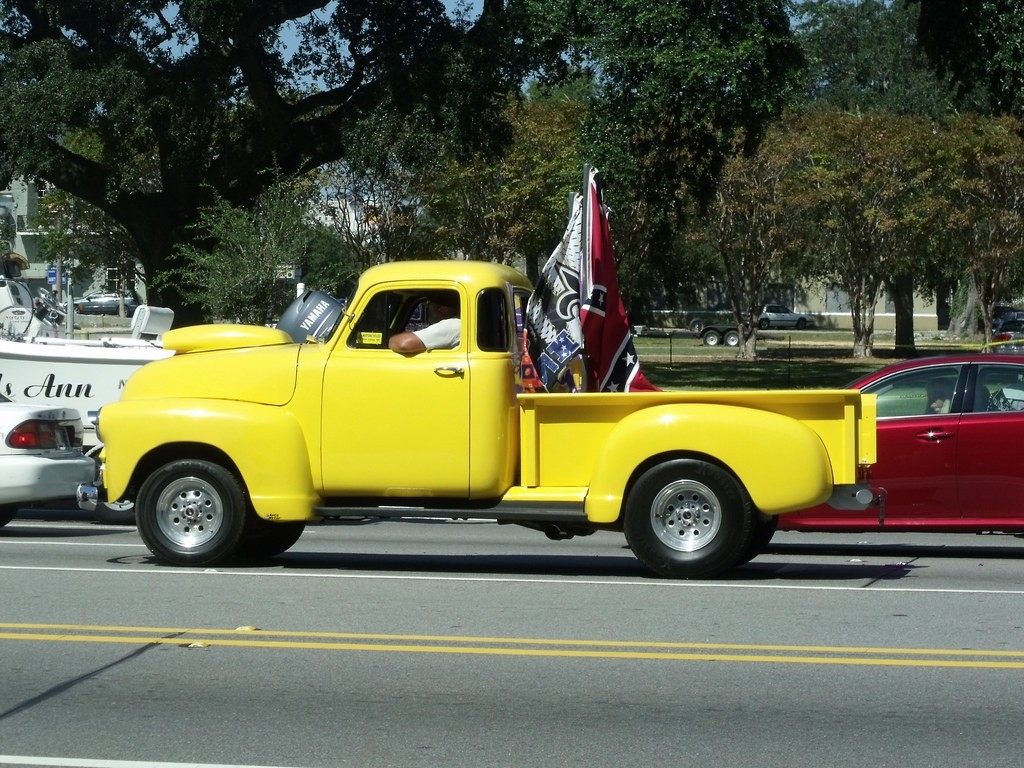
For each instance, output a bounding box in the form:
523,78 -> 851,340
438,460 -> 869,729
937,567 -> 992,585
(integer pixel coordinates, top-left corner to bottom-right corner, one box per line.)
0,275 -> 174,523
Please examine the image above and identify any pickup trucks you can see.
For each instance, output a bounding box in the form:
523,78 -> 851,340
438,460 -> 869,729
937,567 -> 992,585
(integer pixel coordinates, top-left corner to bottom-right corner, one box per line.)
73,257 -> 877,581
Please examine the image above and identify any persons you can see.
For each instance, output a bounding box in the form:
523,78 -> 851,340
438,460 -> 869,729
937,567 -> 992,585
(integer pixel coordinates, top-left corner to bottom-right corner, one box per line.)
925,377 -> 955,414
389,293 -> 461,354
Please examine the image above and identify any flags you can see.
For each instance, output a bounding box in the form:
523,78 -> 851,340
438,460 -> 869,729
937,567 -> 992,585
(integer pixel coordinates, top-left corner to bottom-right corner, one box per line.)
519,162 -> 667,393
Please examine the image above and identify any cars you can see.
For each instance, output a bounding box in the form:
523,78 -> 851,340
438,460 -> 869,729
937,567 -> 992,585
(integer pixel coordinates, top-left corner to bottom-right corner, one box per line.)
0,403 -> 97,530
755,303 -> 815,329
773,355 -> 1024,535
987,305 -> 1024,354
62,288 -> 139,317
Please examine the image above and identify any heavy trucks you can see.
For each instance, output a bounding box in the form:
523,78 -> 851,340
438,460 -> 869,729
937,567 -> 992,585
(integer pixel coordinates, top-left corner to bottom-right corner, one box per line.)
627,279 -> 745,346
